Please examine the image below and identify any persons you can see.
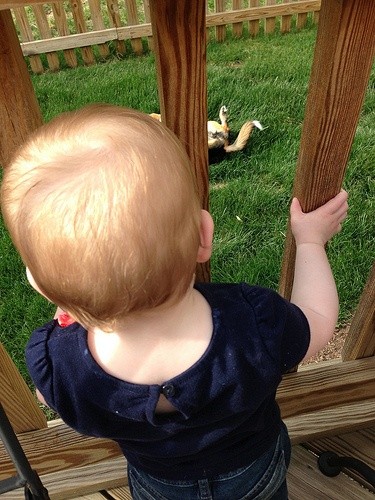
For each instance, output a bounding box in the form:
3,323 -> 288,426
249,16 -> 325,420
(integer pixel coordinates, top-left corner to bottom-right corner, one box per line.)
0,104 -> 349,500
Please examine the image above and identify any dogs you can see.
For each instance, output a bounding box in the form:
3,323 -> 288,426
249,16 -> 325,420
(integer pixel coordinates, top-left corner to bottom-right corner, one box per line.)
148,105 -> 267,153
